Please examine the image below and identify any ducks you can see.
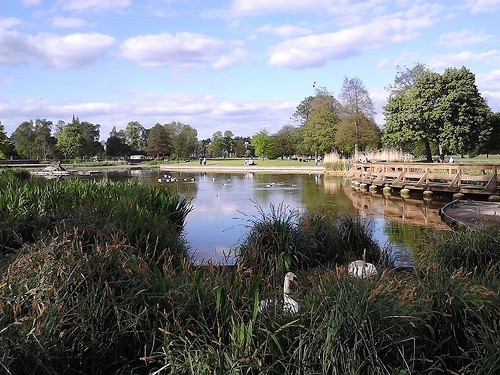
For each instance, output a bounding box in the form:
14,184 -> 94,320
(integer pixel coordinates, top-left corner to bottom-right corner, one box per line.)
291,183 -> 297,187
348,260 -> 378,278
158,175 -> 195,183
267,184 -> 273,187
258,271 -> 300,316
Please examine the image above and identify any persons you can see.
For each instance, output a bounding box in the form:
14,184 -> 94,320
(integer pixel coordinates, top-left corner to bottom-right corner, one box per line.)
359,153 -> 368,163
439,144 -> 444,161
204,156 -> 206,165
199,156 -> 203,164
448,157 -> 454,163
316,157 -> 320,166
245,158 -> 254,166
